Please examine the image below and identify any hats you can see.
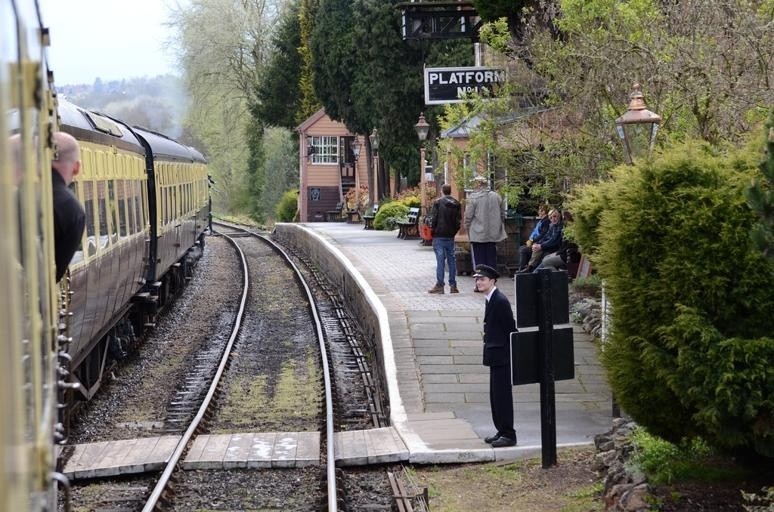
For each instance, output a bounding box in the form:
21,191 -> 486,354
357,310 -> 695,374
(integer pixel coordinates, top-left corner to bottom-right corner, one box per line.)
473,264 -> 500,279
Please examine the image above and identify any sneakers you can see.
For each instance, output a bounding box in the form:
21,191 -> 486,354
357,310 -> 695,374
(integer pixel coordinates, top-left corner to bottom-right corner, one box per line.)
485,434 -> 516,447
428,285 -> 458,294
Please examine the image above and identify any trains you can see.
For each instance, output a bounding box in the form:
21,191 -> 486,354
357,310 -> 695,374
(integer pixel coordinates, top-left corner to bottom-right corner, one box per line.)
0,0 -> 210,510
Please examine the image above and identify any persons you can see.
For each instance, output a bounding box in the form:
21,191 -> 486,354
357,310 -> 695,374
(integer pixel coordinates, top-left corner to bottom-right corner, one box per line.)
516,210 -> 563,274
472,263 -> 519,447
428,183 -> 462,293
519,204 -> 551,270
465,174 -> 509,293
531,209 -> 582,282
7,131 -> 39,269
50,130 -> 86,285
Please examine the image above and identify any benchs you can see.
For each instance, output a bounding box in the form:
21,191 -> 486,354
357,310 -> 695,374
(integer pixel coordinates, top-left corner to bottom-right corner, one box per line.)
345,201 -> 361,224
396,206 -> 421,240
363,204 -> 379,230
325,202 -> 344,222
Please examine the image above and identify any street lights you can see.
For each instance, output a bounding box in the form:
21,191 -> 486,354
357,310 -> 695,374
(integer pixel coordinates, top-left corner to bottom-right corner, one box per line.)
351,132 -> 363,212
617,80 -> 661,167
412,111 -> 431,219
369,128 -> 381,217
